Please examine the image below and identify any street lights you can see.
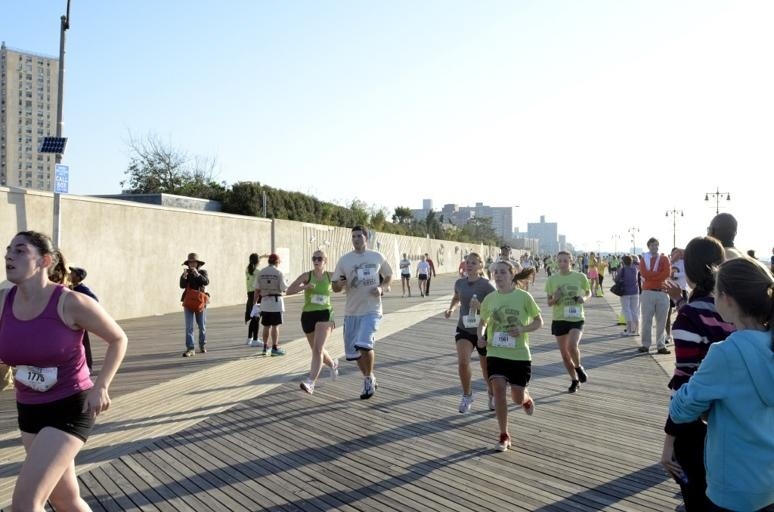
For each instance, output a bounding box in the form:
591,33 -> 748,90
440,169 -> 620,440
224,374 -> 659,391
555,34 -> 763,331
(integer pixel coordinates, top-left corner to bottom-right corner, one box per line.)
663,206 -> 688,250
627,224 -> 640,255
502,205 -> 520,244
704,187 -> 732,219
611,230 -> 620,253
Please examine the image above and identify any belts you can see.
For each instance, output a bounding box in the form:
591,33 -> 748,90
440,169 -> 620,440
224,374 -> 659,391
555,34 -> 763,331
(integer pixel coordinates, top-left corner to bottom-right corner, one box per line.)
651,289 -> 661,291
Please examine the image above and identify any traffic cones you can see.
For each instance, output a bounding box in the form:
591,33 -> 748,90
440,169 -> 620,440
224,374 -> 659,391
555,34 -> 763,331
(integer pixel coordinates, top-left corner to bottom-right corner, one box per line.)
617,308 -> 627,326
596,284 -> 603,296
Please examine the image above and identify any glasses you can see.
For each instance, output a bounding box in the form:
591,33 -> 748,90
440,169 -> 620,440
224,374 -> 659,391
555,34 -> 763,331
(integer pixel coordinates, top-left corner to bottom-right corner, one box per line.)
312,256 -> 322,262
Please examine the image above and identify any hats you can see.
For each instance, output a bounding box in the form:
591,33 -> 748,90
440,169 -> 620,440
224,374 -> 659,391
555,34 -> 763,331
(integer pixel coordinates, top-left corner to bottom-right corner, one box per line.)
269,254 -> 279,262
182,253 -> 205,267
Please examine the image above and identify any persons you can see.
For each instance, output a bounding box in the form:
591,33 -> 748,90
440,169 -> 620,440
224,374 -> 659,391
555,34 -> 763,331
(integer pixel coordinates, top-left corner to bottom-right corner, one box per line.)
283,250 -> 339,396
64,264 -> 100,377
242,252 -> 266,348
487,244 -> 560,291
328,224 -> 393,399
0,229 -> 129,511
414,255 -> 430,298
663,237 -> 773,512
615,239 -> 686,354
458,256 -> 468,278
177,252 -> 210,356
545,252 -> 591,392
571,252 -> 622,296
423,253 -> 435,296
398,253 -> 412,297
703,212 -> 772,284
477,260 -> 543,451
444,253 -> 496,414
250,253 -> 286,356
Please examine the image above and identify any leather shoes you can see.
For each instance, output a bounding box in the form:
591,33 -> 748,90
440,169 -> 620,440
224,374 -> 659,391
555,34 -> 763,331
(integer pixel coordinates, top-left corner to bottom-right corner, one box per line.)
638,347 -> 649,352
658,348 -> 670,354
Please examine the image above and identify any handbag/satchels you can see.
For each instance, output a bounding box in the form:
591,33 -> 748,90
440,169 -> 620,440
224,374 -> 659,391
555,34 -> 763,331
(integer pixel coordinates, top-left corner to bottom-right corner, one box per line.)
611,266 -> 625,296
182,288 -> 209,313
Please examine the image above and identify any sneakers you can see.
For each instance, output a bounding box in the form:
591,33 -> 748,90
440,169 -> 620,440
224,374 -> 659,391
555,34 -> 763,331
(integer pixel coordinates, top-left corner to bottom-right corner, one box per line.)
458,391 -> 474,413
495,434 -> 512,452
330,358 -> 338,381
523,387 -> 535,415
568,380 -> 580,392
183,348 -> 195,357
300,381 -> 313,395
363,373 -> 376,395
488,387 -> 495,410
200,345 -> 206,352
247,338 -> 286,356
360,383 -> 377,399
575,365 -> 588,383
